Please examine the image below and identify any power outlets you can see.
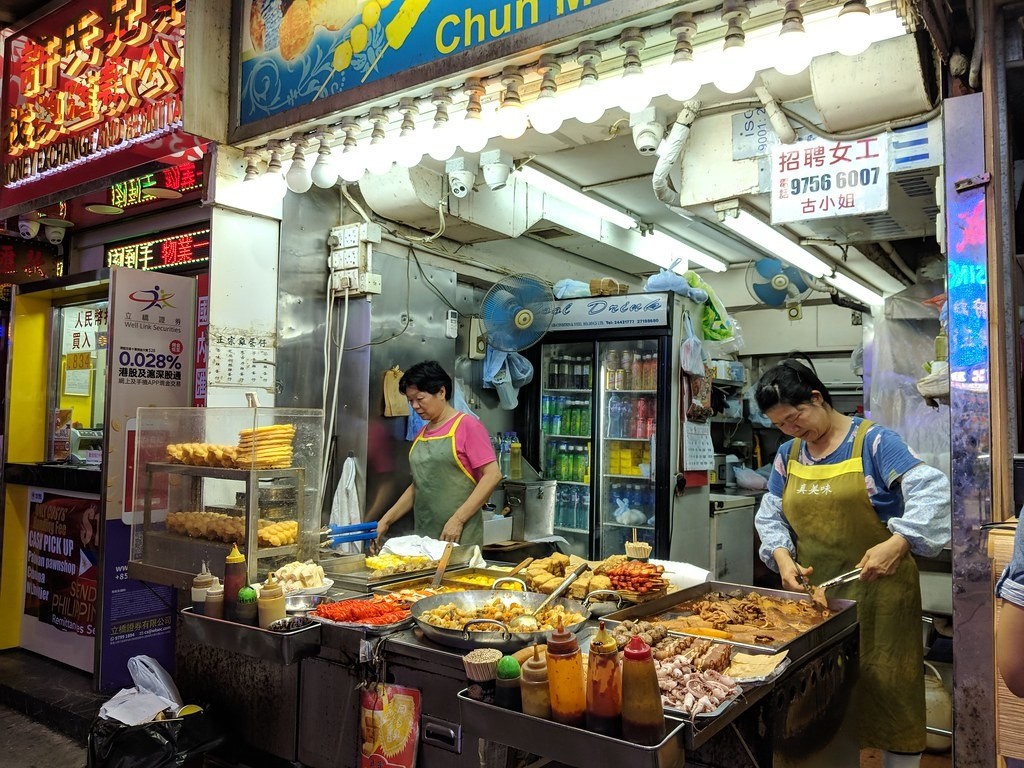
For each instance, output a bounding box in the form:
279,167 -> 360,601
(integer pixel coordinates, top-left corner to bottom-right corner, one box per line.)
328,223 -> 362,291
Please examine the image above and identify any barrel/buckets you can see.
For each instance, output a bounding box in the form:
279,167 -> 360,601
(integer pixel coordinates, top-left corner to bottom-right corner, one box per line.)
726,462 -> 741,483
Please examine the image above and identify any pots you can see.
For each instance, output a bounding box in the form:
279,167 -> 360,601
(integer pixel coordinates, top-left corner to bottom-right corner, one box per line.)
409,577 -> 591,652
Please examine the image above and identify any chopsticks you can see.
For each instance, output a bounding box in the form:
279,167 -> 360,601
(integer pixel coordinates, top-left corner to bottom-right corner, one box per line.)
462,649 -> 502,680
625,541 -> 652,558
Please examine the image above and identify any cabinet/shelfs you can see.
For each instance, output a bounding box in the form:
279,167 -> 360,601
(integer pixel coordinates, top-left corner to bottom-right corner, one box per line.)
706,377 -> 751,422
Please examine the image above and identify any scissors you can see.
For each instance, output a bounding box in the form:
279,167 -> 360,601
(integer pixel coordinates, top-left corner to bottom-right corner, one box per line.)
791,559 -> 825,613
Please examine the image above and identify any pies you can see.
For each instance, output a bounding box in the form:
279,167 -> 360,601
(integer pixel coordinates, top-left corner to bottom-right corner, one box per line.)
234,423 -> 295,470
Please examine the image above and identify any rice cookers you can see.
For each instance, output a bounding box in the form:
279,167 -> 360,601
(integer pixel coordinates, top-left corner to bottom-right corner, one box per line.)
710,454 -> 727,489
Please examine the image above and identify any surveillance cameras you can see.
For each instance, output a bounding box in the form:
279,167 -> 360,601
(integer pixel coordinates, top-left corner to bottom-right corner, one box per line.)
19,220 -> 40,239
45,225 -> 65,244
628,105 -> 667,156
445,157 -> 479,198
479,150 -> 514,192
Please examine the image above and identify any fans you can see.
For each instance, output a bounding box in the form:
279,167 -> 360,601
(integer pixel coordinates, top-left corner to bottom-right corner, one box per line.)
469,272 -> 555,359
745,252 -> 817,308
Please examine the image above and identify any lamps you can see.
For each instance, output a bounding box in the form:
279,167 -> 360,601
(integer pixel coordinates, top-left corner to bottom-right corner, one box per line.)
513,161 -> 887,305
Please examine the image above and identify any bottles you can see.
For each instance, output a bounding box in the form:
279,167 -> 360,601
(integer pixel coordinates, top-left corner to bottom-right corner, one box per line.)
495,655 -> 522,712
586,621 -> 621,738
204,577 -> 224,619
545,615 -> 586,729
621,634 -> 665,747
935,328 -> 947,362
521,642 -> 551,720
925,675 -> 952,750
491,430 -> 522,480
259,572 -> 286,629
192,562 -> 216,615
237,572 -> 257,626
541,345 -> 656,558
224,542 -> 246,620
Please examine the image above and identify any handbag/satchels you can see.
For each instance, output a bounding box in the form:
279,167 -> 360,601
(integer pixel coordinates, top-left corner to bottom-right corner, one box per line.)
482,337 -> 532,411
681,312 -> 704,376
642,257 -> 709,303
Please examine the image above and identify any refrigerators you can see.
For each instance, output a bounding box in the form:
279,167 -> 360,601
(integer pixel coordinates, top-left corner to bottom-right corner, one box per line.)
523,291 -> 714,575
709,494 -> 756,586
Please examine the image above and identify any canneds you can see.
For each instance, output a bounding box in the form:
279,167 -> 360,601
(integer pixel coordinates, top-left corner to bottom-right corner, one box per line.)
607,348 -> 632,390
629,396 -> 656,440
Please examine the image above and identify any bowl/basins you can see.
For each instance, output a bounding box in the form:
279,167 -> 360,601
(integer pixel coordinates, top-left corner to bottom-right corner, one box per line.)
284,595 -> 335,615
267,617 -> 312,630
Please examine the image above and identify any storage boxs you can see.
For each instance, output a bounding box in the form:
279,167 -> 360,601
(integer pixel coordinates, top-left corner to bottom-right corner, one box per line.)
918,560 -> 953,614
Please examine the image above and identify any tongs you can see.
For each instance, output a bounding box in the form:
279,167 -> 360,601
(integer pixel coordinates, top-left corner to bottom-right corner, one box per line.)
819,568 -> 862,589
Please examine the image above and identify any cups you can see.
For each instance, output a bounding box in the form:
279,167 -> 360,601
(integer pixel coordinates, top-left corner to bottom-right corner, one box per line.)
627,557 -> 649,563
466,677 -> 495,704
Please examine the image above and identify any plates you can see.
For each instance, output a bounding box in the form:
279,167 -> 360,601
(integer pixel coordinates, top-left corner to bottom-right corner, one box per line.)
299,578 -> 334,594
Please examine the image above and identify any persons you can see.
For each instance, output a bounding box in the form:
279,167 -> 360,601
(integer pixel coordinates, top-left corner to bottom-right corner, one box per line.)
996,505 -> 1024,703
366,420 -> 396,524
369,361 -> 502,553
754,360 -> 950,768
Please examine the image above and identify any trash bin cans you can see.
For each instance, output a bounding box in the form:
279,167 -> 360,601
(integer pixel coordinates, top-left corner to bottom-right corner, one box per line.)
88,685 -> 236,768
